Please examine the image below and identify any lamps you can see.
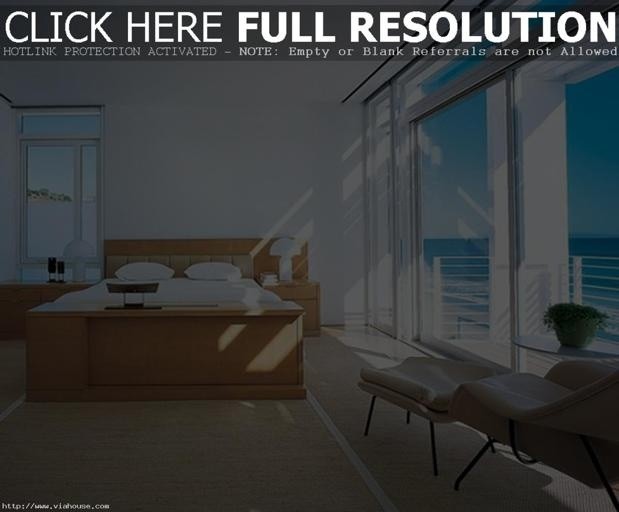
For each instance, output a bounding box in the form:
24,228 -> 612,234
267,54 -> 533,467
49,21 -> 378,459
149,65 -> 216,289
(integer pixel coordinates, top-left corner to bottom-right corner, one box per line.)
268,238 -> 302,281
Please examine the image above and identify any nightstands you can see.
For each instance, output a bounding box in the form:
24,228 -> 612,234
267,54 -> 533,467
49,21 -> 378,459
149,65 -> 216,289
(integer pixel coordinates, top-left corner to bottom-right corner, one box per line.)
257,276 -> 321,337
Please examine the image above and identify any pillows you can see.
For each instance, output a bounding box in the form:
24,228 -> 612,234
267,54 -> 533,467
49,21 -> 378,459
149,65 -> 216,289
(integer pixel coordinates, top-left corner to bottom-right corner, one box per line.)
114,261 -> 243,283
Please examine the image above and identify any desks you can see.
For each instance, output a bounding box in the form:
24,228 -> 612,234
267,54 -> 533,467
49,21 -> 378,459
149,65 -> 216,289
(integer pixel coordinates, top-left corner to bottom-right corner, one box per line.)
24,303 -> 309,402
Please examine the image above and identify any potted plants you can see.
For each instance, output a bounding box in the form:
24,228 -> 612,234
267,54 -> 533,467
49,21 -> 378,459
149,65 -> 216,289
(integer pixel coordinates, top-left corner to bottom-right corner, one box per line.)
542,298 -> 611,349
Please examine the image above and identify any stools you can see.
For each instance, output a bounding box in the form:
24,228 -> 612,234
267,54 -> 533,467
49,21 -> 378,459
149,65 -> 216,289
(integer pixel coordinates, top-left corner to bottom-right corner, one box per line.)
356,356 -> 495,475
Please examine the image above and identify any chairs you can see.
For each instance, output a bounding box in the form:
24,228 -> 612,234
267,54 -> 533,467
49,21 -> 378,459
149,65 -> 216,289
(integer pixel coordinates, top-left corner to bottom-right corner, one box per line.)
446,354 -> 618,510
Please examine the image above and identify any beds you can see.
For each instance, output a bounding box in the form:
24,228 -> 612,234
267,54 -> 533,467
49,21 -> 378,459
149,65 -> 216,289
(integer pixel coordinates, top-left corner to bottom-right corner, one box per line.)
25,237 -> 305,302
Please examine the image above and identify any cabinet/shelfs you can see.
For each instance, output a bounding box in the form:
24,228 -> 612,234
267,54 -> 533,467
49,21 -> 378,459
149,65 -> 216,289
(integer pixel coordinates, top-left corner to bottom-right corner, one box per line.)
0,280 -> 95,340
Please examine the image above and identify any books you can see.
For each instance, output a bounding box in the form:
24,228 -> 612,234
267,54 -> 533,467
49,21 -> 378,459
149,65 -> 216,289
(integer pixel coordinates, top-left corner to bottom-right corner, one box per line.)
259,272 -> 278,284
